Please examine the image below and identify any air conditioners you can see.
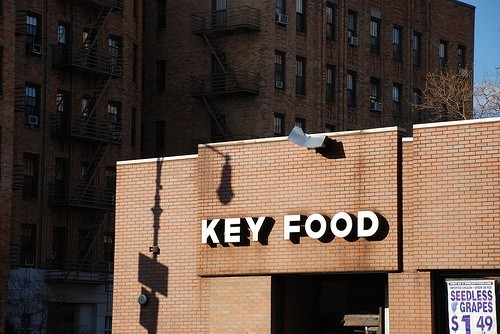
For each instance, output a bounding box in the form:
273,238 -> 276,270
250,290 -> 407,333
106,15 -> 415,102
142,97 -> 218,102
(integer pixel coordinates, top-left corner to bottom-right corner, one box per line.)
33,43 -> 41,54
26,257 -> 34,266
348,36 -> 358,46
276,81 -> 283,88
113,131 -> 122,140
28,115 -> 38,124
371,102 -> 383,112
276,14 -> 288,24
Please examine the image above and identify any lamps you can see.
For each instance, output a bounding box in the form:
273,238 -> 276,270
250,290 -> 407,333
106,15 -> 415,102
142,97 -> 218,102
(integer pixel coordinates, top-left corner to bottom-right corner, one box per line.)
287,126 -> 331,150
